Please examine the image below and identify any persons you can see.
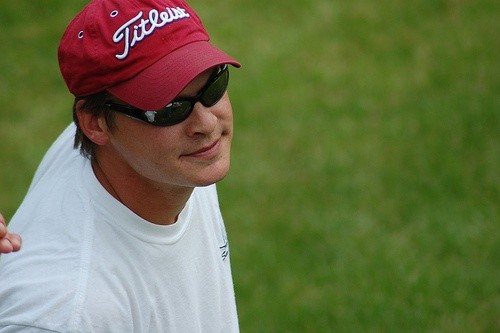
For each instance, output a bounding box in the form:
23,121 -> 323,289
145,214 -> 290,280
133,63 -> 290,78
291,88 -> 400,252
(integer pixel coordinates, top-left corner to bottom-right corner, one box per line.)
0,213 -> 22,254
0,0 -> 239,333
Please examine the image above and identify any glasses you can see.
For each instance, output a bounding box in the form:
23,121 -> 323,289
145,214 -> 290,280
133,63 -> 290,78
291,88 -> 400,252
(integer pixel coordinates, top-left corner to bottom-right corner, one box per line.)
94,63 -> 229,126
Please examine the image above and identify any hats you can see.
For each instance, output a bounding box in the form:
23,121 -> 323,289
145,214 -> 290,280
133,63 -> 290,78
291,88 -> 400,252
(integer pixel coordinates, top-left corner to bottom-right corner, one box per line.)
58,0 -> 242,112
